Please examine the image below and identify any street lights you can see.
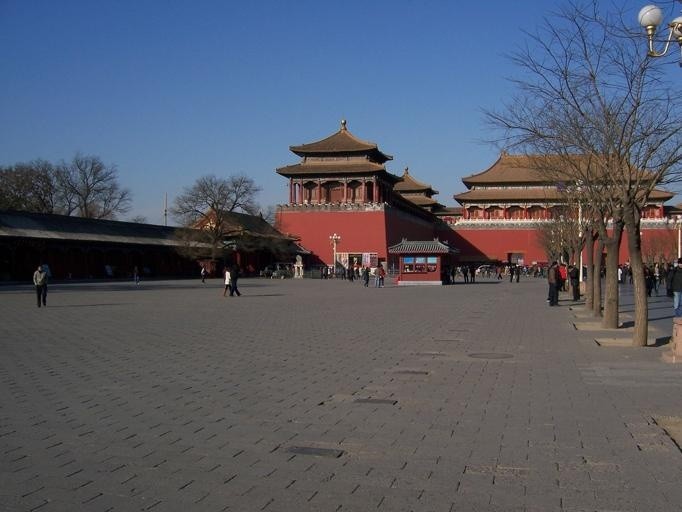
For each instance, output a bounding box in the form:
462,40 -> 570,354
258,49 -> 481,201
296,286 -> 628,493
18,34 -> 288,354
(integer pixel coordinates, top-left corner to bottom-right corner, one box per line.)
566,179 -> 598,283
638,5 -> 682,67
669,215 -> 682,258
329,233 -> 341,273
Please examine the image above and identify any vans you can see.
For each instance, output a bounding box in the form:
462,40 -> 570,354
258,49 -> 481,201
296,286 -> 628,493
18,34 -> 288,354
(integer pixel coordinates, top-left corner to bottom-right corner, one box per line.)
475,265 -> 491,276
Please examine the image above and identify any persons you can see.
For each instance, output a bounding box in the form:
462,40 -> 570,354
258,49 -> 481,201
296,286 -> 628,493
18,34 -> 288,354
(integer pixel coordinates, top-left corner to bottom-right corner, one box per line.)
223,264 -> 242,296
451,258 -> 682,317
320,256 -> 385,288
134,266 -> 140,288
33,266 -> 48,307
201,265 -> 207,282
